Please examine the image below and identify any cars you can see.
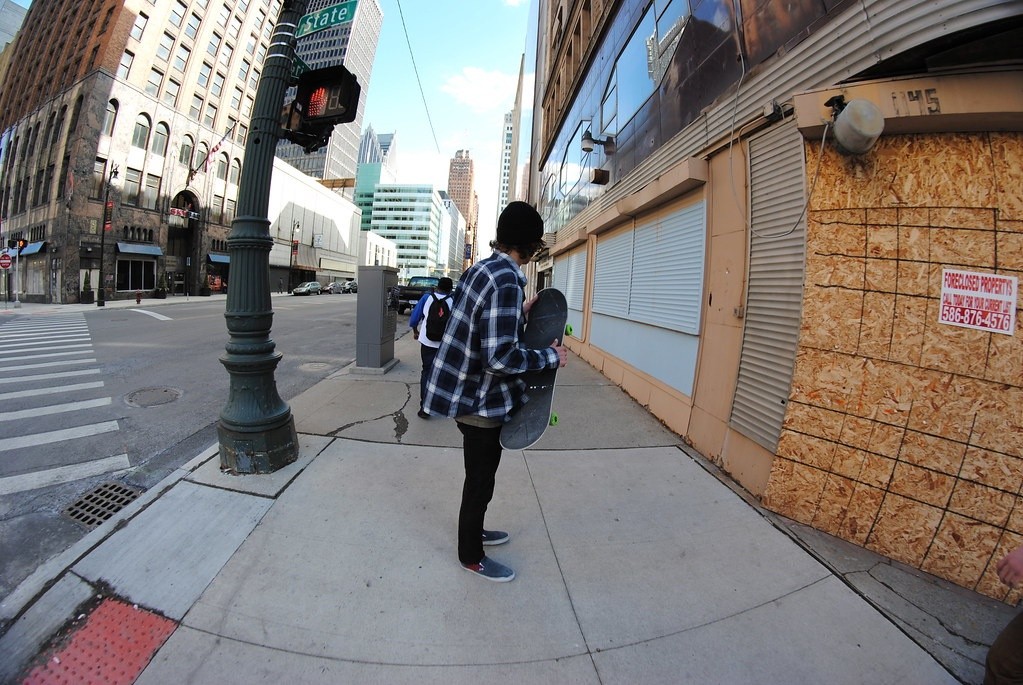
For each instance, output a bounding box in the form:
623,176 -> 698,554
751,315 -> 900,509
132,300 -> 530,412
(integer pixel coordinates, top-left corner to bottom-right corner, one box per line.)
345,281 -> 357,293
293,281 -> 322,295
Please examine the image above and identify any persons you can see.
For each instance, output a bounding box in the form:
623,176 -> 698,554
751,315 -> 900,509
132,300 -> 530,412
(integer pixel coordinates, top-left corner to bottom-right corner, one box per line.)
423,201 -> 568,581
409,277 -> 453,418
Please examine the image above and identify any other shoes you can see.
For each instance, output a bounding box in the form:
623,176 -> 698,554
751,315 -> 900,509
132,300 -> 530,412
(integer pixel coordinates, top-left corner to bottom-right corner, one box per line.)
417,407 -> 430,418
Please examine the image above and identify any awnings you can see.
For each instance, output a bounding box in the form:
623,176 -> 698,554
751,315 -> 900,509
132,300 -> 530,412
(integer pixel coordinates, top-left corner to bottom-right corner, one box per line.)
209,253 -> 230,263
20,241 -> 47,255
117,243 -> 163,255
8,248 -> 20,257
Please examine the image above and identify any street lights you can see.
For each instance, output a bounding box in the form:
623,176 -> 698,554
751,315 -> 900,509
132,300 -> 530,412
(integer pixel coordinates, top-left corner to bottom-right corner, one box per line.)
94,161 -> 119,306
287,219 -> 300,293
466,222 -> 476,265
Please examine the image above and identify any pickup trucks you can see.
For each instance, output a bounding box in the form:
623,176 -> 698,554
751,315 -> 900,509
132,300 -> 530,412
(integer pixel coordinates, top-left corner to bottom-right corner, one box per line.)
398,275 -> 440,315
322,281 -> 346,294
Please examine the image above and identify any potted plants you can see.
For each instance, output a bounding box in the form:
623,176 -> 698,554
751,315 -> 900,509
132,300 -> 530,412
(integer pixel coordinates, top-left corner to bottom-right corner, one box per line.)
80,271 -> 94,305
200,272 -> 212,296
153,271 -> 169,299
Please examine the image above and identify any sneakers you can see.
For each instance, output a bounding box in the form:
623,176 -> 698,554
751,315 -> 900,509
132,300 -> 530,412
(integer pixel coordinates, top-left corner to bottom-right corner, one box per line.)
481,528 -> 509,546
461,557 -> 515,582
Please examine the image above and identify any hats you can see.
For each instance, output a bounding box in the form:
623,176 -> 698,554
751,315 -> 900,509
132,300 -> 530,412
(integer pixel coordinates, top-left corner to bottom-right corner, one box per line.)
497,201 -> 544,246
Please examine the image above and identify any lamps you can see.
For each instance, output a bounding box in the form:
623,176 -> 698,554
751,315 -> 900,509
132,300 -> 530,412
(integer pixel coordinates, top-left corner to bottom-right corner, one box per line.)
581,131 -> 608,152
109,164 -> 120,180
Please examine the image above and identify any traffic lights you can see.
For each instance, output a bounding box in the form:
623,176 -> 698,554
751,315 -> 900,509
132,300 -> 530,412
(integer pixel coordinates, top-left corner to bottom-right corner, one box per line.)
19,240 -> 27,247
294,65 -> 360,123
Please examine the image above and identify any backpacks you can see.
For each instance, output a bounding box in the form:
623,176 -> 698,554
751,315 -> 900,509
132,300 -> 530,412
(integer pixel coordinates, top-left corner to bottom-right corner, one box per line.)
426,292 -> 454,342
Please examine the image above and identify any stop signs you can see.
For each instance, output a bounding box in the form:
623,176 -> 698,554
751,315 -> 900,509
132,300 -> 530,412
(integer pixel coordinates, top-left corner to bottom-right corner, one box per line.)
0,254 -> 11,268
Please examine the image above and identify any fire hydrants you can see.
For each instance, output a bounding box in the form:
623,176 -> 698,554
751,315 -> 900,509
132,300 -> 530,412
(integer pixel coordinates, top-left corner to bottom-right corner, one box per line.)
135,290 -> 142,304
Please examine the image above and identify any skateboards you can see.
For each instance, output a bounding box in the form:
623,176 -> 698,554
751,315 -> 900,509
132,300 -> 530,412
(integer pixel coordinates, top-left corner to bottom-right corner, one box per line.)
498,287 -> 574,453
